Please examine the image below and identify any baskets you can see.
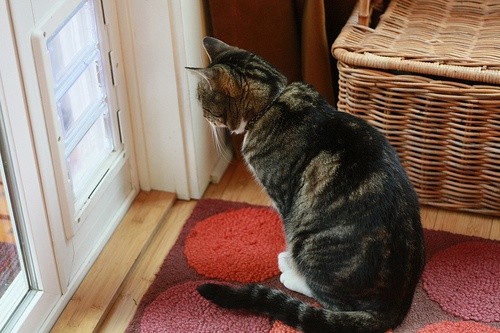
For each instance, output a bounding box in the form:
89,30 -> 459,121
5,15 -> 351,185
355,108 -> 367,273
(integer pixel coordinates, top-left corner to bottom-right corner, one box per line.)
330,0 -> 500,218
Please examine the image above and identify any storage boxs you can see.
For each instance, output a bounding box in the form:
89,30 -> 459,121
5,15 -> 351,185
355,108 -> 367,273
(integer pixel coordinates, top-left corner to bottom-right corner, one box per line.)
330,0 -> 500,218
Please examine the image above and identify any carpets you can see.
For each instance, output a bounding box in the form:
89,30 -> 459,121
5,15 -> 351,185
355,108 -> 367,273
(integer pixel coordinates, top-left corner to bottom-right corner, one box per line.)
123,198 -> 500,333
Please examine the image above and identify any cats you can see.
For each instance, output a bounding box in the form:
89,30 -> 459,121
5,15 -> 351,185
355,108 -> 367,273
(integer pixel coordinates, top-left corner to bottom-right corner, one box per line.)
184,36 -> 425,332
